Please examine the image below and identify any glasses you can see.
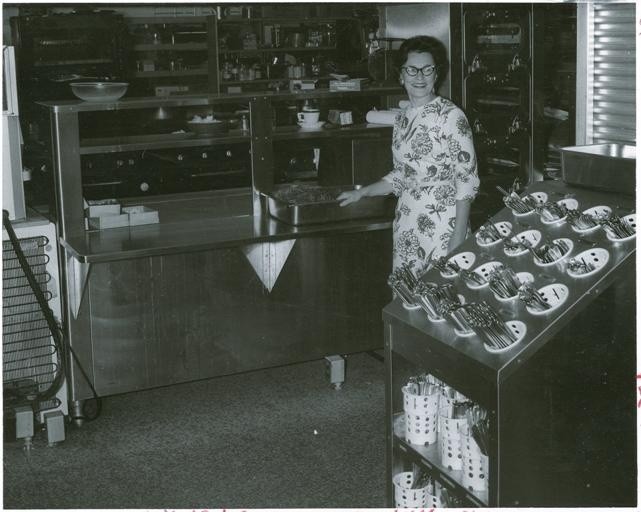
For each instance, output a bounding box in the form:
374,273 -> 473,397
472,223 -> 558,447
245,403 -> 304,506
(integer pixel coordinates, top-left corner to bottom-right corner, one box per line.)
400,65 -> 435,77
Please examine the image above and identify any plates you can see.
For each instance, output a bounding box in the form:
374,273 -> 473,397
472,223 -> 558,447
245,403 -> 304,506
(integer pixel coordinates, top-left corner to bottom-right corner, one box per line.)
298,121 -> 327,129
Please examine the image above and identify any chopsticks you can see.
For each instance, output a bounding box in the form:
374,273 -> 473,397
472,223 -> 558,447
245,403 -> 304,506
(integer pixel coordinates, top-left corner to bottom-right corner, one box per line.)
496,185 -> 635,238
386,263 -> 518,351
409,461 -> 472,507
429,256 -> 552,311
476,220 -> 595,275
405,372 -> 489,456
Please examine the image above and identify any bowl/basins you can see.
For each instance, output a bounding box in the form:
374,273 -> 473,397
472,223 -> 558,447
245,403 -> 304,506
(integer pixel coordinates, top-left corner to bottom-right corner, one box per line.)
69,83 -> 130,102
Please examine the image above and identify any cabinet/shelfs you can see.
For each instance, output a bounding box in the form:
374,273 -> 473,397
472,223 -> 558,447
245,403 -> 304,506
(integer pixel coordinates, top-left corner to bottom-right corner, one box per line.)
13,4 -> 382,228
383,178 -> 637,508
458,3 -> 578,239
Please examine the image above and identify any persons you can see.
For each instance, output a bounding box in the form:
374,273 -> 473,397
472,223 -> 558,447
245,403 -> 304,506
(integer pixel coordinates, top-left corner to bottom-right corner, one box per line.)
335,35 -> 480,302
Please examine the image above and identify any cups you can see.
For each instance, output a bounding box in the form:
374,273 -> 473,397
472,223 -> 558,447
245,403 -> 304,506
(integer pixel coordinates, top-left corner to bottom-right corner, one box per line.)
297,109 -> 320,124
